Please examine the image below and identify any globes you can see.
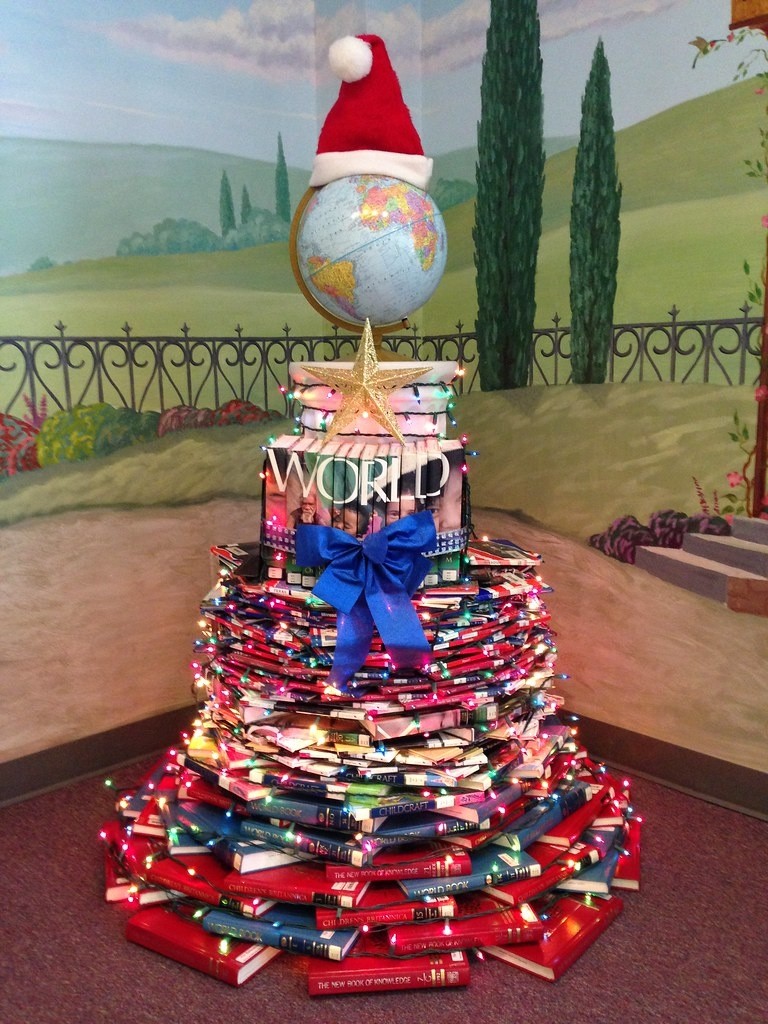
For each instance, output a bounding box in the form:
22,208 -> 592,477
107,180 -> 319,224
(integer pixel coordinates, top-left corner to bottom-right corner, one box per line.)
289,177 -> 450,359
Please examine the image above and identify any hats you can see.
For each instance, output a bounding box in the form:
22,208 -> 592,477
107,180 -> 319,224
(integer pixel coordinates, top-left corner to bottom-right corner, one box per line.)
309,35 -> 434,191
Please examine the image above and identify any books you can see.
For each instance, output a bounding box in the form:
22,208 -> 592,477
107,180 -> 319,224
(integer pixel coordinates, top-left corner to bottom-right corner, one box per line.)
323,842 -> 473,884
100,539 -> 643,996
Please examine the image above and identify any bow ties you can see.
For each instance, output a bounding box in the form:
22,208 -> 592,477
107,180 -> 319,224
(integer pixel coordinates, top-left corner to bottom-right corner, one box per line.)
296,514 -> 436,687
